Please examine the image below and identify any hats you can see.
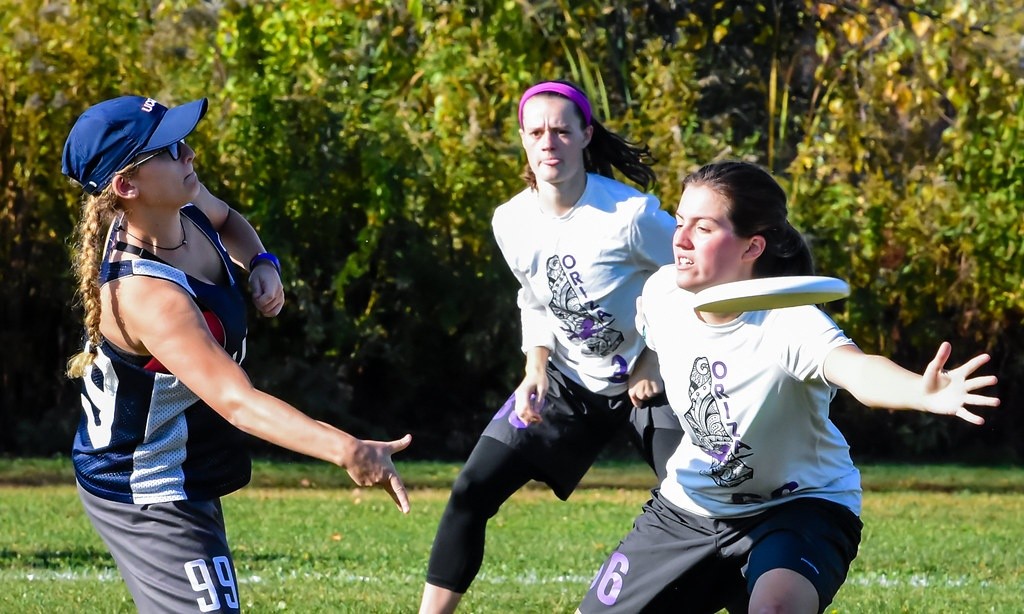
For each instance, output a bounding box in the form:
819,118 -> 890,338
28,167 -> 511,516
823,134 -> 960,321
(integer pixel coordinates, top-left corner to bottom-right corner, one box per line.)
61,96 -> 208,195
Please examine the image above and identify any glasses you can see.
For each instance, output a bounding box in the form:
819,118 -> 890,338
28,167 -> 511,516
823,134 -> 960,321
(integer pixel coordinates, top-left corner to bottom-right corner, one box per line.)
115,137 -> 186,175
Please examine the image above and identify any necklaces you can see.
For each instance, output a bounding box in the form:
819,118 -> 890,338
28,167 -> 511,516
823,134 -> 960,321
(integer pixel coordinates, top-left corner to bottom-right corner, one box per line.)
117,218 -> 187,251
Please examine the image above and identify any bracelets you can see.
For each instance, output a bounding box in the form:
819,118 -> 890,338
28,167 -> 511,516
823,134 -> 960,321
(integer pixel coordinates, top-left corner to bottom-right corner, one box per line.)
249,252 -> 281,276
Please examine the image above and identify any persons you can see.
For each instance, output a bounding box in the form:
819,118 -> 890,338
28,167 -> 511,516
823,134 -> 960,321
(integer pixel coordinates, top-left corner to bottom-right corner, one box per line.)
57,95 -> 412,613
414,77 -> 686,614
569,164 -> 1003,614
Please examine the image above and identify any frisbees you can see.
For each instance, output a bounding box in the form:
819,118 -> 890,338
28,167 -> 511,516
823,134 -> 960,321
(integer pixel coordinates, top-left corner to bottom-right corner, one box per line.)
694,273 -> 852,313
